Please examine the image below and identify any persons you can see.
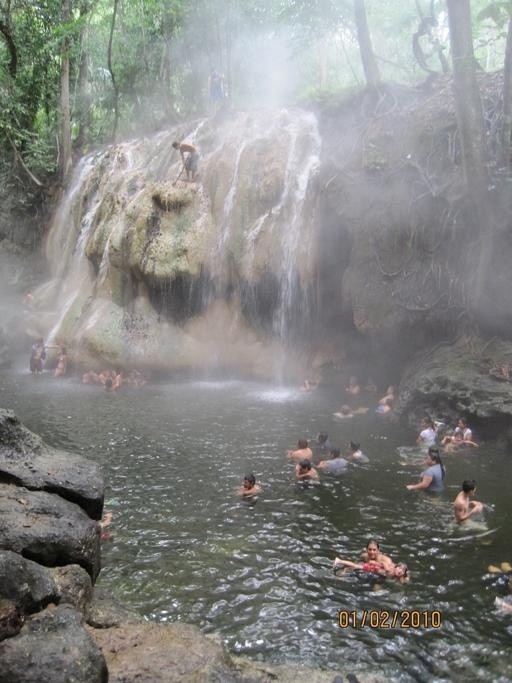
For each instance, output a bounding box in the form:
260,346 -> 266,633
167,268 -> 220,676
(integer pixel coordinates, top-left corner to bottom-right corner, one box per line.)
171,139 -> 200,182
407,445 -> 447,498
454,479 -> 482,524
289,431 -> 369,482
28,338 -> 147,391
238,473 -> 264,498
416,415 -> 478,455
100,512 -> 114,540
335,537 -> 407,584
